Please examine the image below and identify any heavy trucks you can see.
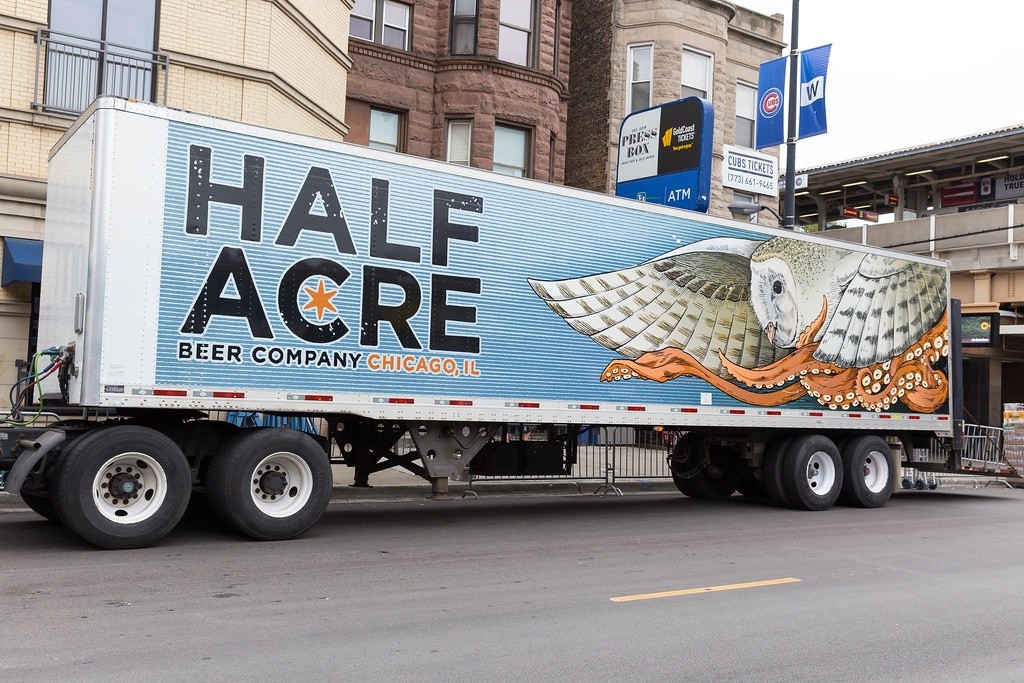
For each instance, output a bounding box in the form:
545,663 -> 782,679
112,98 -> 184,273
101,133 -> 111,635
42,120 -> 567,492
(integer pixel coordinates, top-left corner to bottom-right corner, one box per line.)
2,94 -> 953,550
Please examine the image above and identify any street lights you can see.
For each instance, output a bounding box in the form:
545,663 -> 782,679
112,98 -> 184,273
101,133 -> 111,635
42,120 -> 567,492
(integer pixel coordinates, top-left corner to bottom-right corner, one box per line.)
728,201 -> 798,231
